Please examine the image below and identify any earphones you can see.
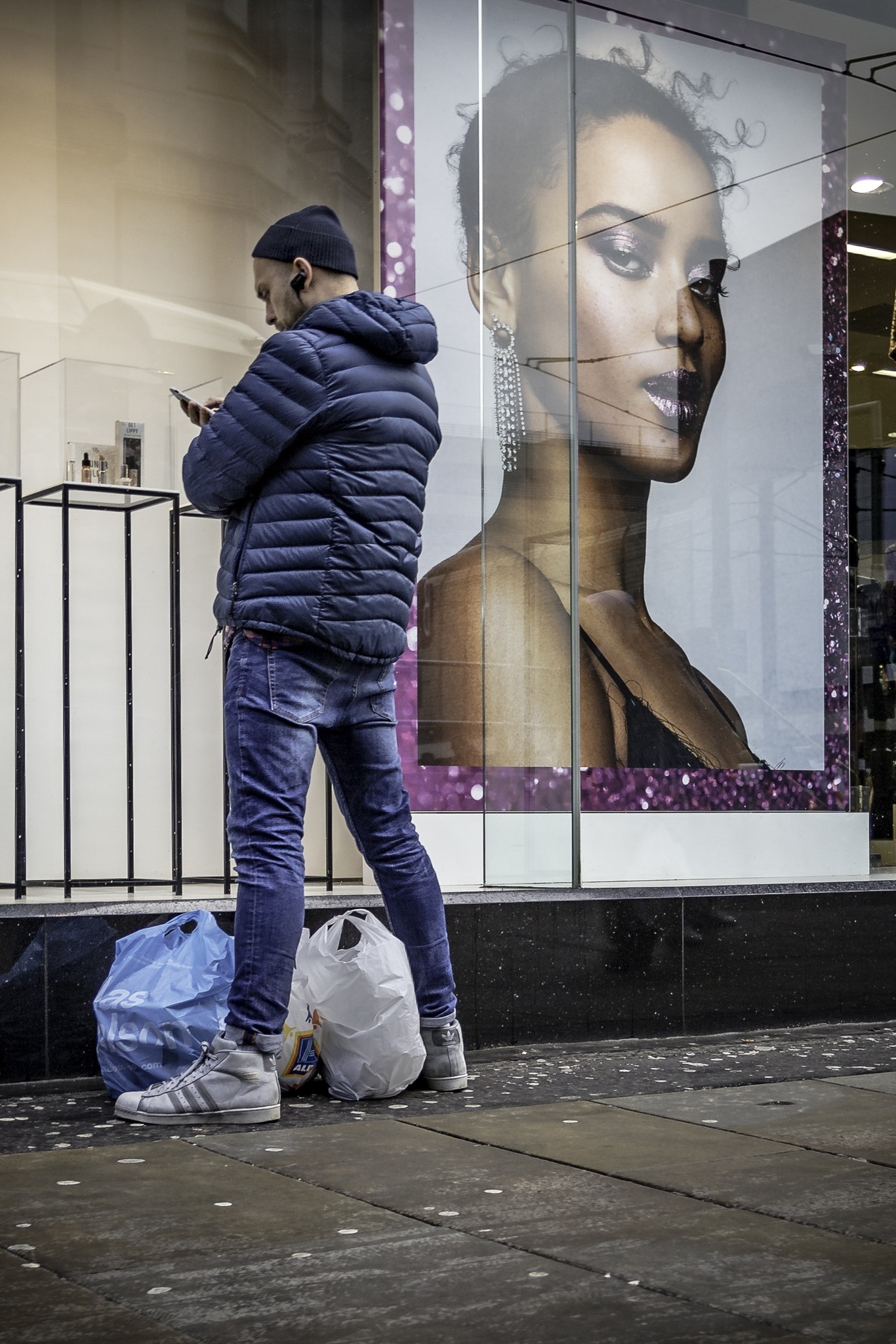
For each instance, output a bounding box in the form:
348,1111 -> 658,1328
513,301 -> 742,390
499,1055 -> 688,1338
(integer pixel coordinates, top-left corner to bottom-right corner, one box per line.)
290,273 -> 307,291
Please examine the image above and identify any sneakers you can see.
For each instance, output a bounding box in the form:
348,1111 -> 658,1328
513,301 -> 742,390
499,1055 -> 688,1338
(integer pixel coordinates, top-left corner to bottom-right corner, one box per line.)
421,1018 -> 468,1092
113,1031 -> 282,1126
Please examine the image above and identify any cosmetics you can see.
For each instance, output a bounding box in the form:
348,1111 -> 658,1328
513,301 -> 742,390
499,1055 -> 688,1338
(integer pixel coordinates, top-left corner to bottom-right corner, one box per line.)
119,464 -> 132,486
81,452 -> 92,483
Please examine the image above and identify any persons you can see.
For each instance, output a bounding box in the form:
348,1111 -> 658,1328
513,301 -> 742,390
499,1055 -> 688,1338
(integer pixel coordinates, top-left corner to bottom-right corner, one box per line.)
415,52 -> 774,769
114,204 -> 469,1125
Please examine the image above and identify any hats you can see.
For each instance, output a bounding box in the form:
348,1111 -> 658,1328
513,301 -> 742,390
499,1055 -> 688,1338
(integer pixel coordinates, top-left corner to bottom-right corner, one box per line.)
252,204 -> 361,281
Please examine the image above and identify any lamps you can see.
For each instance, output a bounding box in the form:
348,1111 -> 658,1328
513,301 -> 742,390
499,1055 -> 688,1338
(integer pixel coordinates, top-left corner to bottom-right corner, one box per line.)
872,367 -> 896,377
851,361 -> 866,371
847,243 -> 896,260
848,163 -> 883,194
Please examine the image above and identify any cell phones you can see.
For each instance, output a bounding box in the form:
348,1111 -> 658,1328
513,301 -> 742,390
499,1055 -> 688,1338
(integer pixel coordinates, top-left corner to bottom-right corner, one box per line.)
171,387 -> 217,417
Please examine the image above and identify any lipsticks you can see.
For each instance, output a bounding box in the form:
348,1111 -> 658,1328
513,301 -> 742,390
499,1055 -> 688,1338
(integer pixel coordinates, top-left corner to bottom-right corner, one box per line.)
98,454 -> 106,484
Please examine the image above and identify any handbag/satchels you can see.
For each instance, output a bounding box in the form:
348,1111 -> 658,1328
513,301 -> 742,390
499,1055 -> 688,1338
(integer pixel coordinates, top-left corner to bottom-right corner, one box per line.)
301,907 -> 427,1101
273,927 -> 326,1092
94,909 -> 235,1099
